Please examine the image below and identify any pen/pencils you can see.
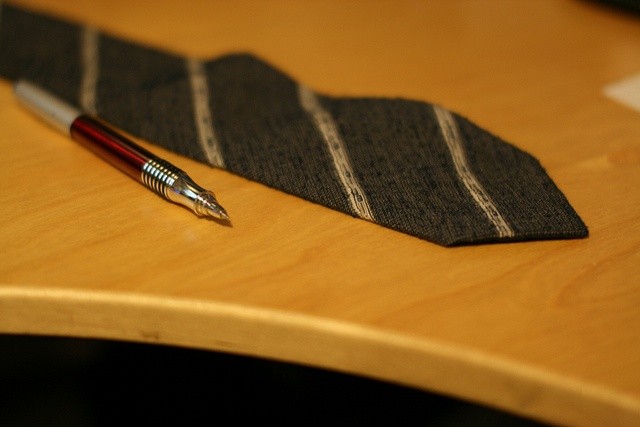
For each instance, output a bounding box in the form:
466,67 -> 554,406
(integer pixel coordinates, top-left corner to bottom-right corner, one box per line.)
14,78 -> 232,228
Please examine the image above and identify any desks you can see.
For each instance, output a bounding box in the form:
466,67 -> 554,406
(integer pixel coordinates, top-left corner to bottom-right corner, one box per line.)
0,0 -> 639,425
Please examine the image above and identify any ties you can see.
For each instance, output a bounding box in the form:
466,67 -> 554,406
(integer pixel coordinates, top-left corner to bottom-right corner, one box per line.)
0,5 -> 589,248
